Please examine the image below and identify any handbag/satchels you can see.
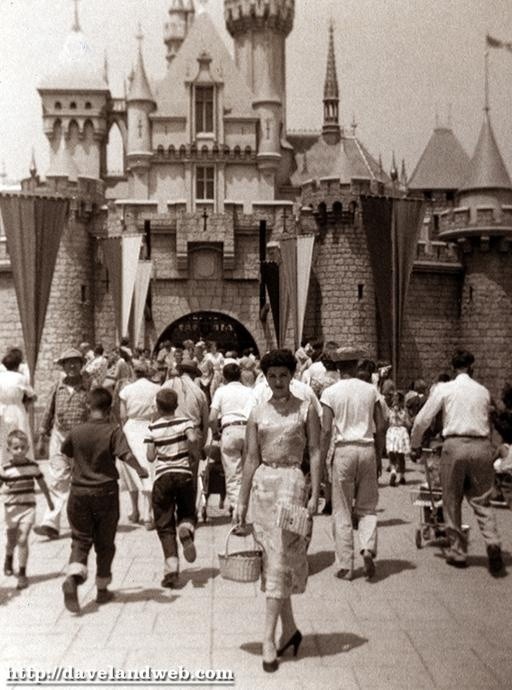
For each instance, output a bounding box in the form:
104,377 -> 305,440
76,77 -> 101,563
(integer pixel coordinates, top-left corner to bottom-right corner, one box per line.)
277,503 -> 309,537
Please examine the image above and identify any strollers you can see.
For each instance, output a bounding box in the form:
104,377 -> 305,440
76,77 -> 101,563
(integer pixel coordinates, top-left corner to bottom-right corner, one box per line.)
196,434 -> 232,523
408,448 -> 470,554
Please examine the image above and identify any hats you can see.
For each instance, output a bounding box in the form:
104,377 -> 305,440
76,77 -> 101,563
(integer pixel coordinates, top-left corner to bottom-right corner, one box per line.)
332,347 -> 359,361
175,360 -> 202,376
56,348 -> 85,364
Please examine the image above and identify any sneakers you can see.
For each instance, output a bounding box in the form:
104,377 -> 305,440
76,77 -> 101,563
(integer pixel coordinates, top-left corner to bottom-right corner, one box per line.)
363,552 -> 375,579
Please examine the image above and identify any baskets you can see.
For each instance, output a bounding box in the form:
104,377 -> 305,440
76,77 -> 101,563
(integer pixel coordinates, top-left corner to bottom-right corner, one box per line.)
219,522 -> 265,583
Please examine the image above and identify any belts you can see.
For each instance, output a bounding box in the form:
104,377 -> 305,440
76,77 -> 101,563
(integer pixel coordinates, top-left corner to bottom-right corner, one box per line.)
444,432 -> 490,440
220,420 -> 249,428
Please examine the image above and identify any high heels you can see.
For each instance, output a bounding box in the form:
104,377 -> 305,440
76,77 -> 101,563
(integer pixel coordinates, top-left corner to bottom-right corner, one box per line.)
273,630 -> 303,659
261,657 -> 281,672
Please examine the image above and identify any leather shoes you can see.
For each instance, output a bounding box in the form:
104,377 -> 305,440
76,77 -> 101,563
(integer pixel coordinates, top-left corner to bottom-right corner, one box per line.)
161,574 -> 180,588
17,576 -> 28,590
33,525 -> 59,540
4,563 -> 13,575
487,545 -> 505,577
97,588 -> 114,601
62,578 -> 79,611
179,528 -> 196,563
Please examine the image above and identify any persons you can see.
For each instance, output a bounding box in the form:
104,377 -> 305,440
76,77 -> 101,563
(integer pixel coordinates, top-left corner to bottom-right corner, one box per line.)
2,337 -> 512,672
